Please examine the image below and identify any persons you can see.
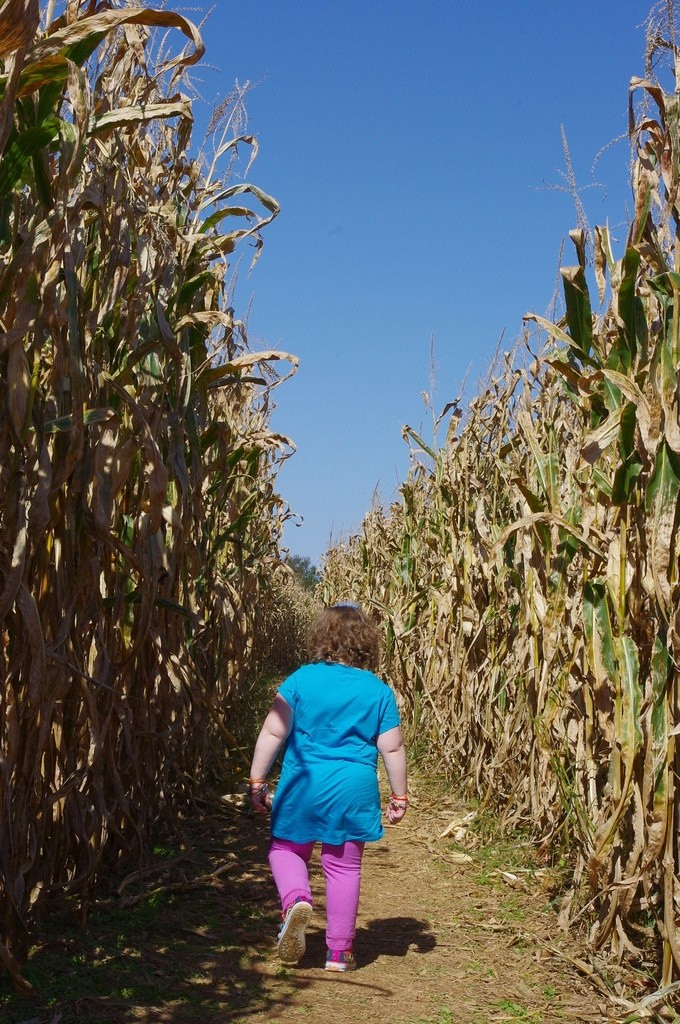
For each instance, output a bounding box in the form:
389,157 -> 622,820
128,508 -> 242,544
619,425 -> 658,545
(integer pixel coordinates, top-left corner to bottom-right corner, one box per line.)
248,602 -> 408,973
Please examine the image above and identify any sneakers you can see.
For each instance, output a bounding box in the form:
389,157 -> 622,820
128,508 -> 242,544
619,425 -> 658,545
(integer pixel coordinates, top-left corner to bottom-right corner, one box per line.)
323,945 -> 357,971
277,896 -> 313,961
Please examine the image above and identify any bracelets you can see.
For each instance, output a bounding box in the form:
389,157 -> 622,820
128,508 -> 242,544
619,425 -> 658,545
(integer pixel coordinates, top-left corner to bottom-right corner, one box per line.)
389,794 -> 409,808
248,779 -> 267,796
